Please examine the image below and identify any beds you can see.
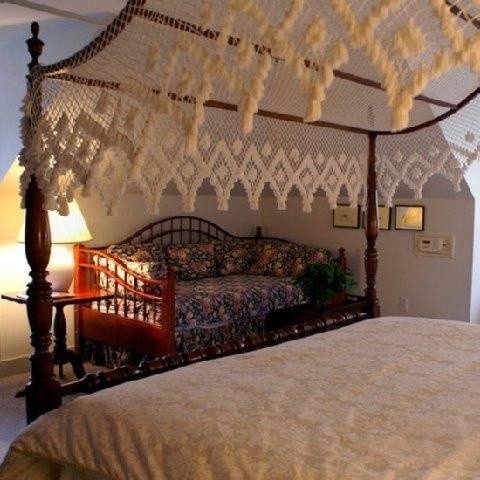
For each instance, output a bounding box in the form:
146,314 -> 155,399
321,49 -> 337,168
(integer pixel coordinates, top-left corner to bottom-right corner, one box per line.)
0,21 -> 480,480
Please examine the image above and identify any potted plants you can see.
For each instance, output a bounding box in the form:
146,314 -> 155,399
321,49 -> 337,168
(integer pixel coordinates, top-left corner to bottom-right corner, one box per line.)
292,261 -> 361,314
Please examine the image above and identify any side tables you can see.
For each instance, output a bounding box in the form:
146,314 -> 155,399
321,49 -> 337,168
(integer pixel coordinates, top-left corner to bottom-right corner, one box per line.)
2,287 -> 114,398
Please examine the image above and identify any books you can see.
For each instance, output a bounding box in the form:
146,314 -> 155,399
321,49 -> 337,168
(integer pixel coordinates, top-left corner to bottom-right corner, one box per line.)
17,291 -> 77,303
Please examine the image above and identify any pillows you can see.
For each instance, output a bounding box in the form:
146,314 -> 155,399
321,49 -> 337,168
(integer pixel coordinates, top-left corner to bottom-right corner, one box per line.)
92,239 -> 329,301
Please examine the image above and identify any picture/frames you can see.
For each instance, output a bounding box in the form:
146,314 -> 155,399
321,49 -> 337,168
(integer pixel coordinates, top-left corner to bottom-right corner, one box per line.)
395,204 -> 424,230
362,205 -> 391,229
333,203 -> 360,228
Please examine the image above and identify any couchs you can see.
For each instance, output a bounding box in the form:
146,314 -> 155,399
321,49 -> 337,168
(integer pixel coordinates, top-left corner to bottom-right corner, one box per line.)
73,215 -> 348,360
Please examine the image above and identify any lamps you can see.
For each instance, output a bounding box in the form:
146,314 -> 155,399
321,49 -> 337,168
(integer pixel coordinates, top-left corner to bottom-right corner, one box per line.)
18,193 -> 93,292
